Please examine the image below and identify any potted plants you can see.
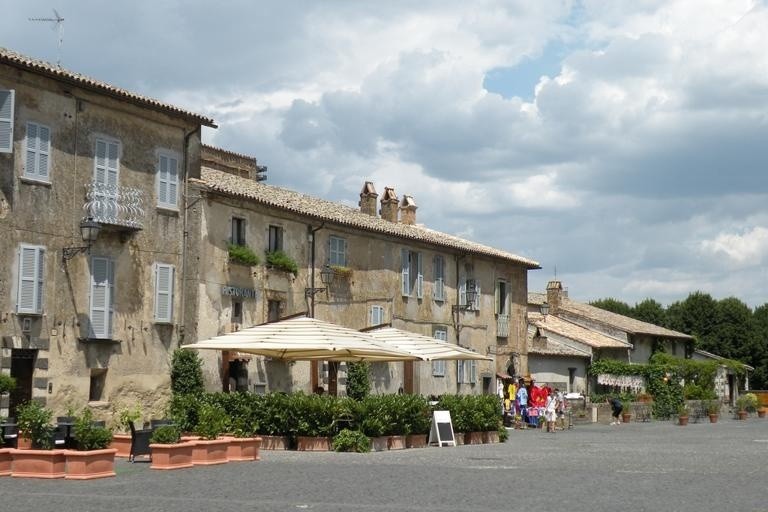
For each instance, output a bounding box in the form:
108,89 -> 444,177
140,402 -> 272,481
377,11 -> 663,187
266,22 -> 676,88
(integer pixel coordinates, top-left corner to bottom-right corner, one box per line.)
0,374 -> 508,481
621,400 -> 633,423
678,399 -> 766,425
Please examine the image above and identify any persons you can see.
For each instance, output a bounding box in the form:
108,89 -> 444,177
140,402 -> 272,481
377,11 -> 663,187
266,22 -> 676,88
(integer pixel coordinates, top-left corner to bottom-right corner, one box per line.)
497,376 -> 568,433
606,395 -> 624,425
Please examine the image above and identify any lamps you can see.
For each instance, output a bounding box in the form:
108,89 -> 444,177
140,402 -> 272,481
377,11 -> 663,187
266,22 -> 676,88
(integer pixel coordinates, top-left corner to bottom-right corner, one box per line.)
525,301 -> 549,325
304,263 -> 335,300
451,285 -> 478,315
62,216 -> 102,265
